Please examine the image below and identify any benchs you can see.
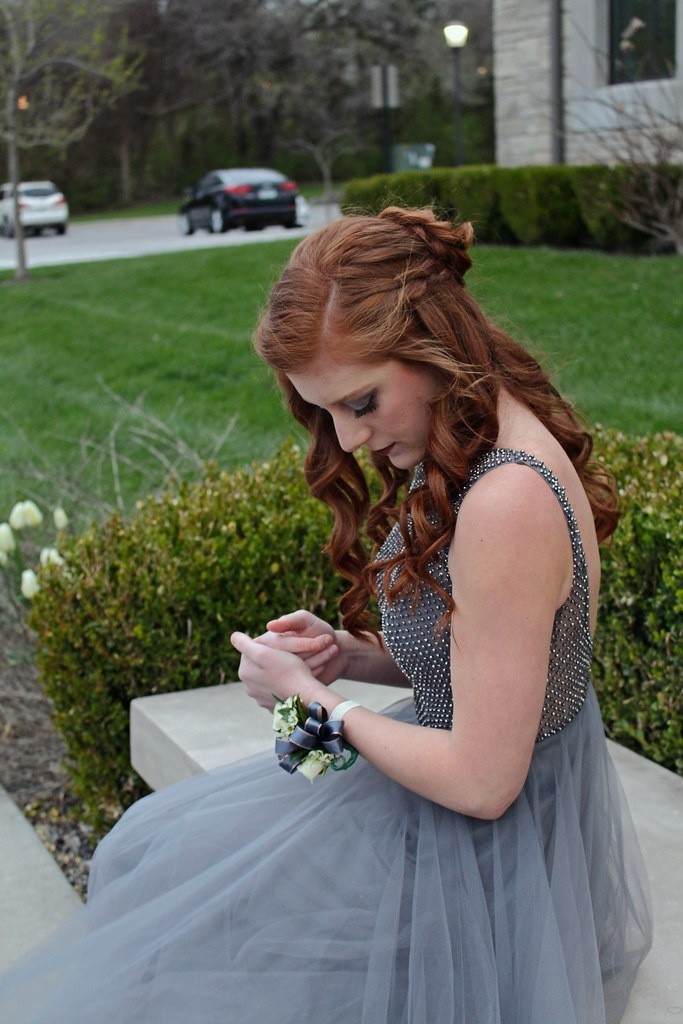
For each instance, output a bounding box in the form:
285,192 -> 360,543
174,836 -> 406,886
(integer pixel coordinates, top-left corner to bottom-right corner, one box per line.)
130,681 -> 682,1024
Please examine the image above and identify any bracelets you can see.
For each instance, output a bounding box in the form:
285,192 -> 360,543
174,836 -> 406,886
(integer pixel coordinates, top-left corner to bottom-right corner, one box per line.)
266,691 -> 360,782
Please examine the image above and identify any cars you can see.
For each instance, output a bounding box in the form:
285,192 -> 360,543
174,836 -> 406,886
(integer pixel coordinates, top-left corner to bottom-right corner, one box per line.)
176,167 -> 307,237
0,178 -> 69,240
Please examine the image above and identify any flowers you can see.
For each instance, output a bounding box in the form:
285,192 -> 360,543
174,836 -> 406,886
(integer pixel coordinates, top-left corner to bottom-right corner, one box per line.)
270,691 -> 357,781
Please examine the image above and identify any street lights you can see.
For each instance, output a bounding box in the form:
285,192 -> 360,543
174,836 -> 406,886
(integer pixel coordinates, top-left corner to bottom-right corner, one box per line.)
443,19 -> 470,166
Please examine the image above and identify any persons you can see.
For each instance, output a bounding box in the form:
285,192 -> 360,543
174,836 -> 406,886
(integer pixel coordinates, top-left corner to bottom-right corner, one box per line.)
137,204 -> 607,1023
